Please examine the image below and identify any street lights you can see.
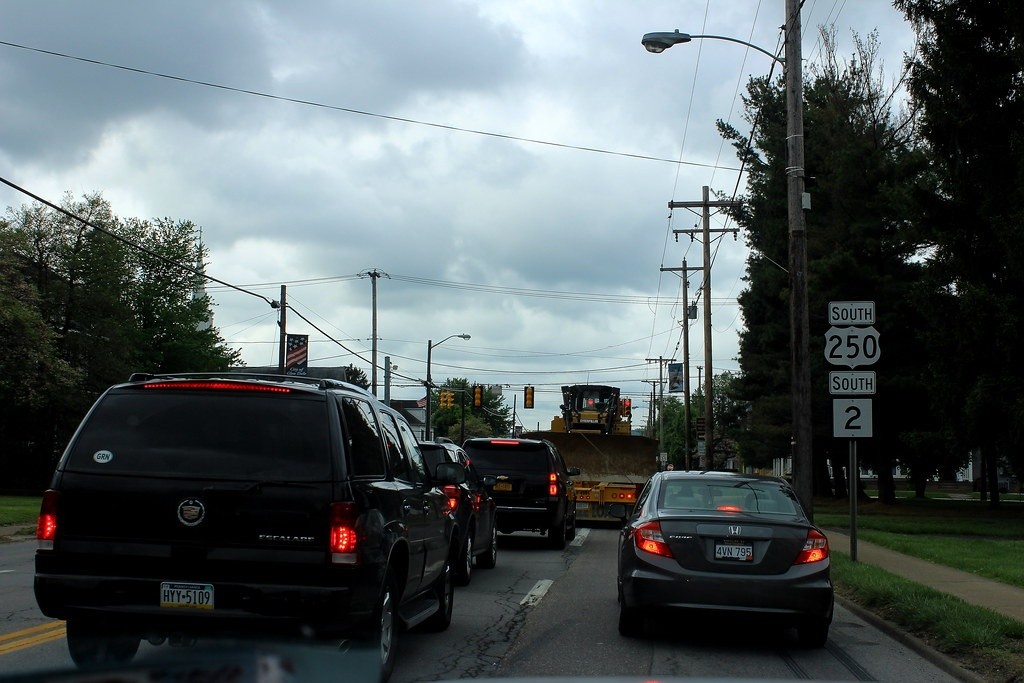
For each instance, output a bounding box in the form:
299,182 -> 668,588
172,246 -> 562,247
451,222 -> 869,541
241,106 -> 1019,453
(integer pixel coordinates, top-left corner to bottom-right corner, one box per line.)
424,333 -> 477,445
639,30 -> 815,533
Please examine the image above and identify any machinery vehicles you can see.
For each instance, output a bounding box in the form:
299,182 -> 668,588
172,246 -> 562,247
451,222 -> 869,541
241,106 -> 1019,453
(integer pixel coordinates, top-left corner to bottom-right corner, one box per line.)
515,383 -> 662,483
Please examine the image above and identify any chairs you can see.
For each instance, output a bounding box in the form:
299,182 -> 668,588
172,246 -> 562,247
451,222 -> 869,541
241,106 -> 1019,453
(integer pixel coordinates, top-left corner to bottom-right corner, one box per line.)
752,499 -> 779,512
674,496 -> 701,509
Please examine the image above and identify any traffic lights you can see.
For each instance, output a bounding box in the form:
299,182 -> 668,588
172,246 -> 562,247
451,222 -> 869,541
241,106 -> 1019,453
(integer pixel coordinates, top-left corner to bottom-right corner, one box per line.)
441,393 -> 455,409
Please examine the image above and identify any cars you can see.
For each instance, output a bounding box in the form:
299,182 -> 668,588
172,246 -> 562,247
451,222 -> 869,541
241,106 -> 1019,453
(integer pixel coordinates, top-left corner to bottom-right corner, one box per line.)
616,468 -> 836,652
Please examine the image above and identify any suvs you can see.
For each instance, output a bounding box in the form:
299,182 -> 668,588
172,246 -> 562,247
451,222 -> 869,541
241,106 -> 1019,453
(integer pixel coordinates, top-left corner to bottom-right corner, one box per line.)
416,435 -> 498,587
33,371 -> 460,677
462,436 -> 581,550
